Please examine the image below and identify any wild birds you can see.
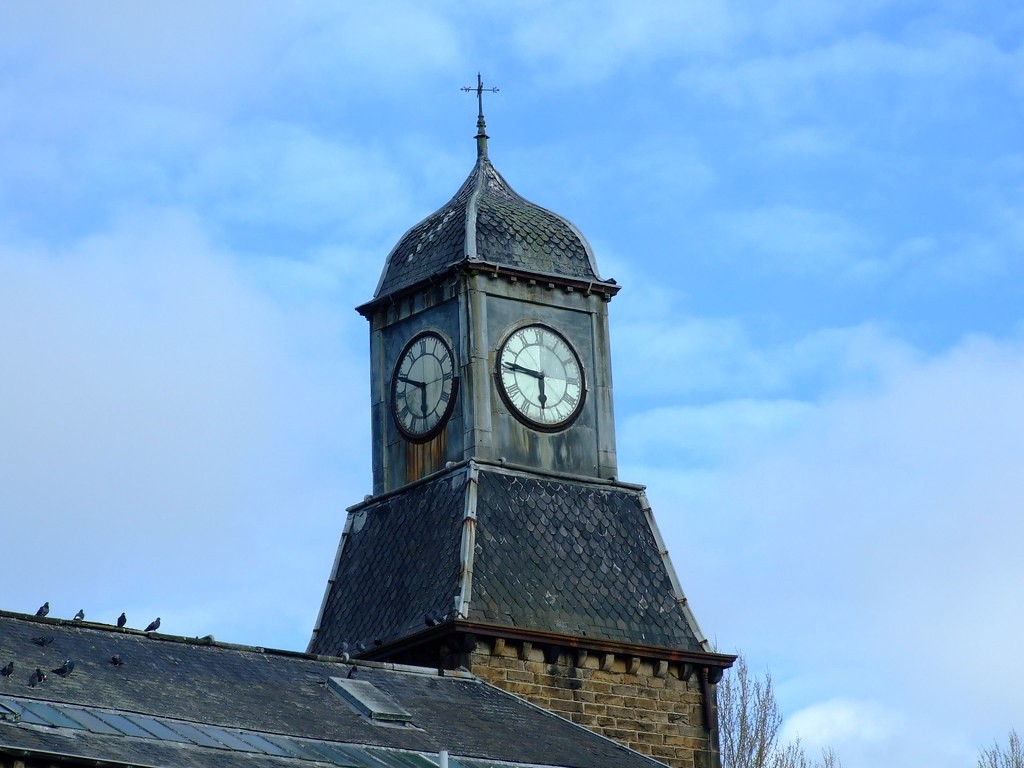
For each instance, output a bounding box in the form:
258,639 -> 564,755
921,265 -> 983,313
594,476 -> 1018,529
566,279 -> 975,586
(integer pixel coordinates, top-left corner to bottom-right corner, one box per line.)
117,612 -> 127,629
34,602 -> 50,618
0,661 -> 15,678
31,635 -> 56,648
51,659 -> 75,678
422,609 -> 445,628
27,669 -> 47,688
112,653 -> 124,668
72,609 -> 84,622
355,637 -> 383,653
347,664 -> 359,679
144,617 -> 161,633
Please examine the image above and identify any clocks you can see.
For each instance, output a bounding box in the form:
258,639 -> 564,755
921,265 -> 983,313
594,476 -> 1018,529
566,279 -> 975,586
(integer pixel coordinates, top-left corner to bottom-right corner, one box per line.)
390,327 -> 459,444
494,318 -> 588,433
